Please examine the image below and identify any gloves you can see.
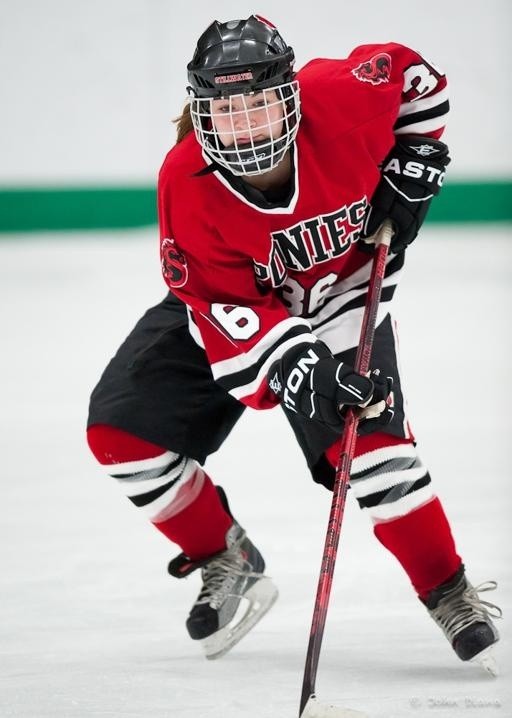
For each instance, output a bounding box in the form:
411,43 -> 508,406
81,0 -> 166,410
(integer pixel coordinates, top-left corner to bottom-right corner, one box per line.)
360,135 -> 451,254
265,338 -> 394,436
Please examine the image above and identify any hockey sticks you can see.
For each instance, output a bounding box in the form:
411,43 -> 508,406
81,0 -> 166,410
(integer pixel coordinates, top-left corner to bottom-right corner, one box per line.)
297,219 -> 401,717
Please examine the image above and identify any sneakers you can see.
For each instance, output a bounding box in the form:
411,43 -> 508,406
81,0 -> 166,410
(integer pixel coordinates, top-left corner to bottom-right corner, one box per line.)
167,486 -> 266,640
418,562 -> 499,660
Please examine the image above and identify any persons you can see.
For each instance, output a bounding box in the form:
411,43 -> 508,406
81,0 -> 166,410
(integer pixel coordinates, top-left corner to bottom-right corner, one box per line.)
85,15 -> 505,662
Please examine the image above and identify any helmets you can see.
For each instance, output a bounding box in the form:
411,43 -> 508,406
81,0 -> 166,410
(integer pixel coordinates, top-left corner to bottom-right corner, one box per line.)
185,13 -> 302,177
